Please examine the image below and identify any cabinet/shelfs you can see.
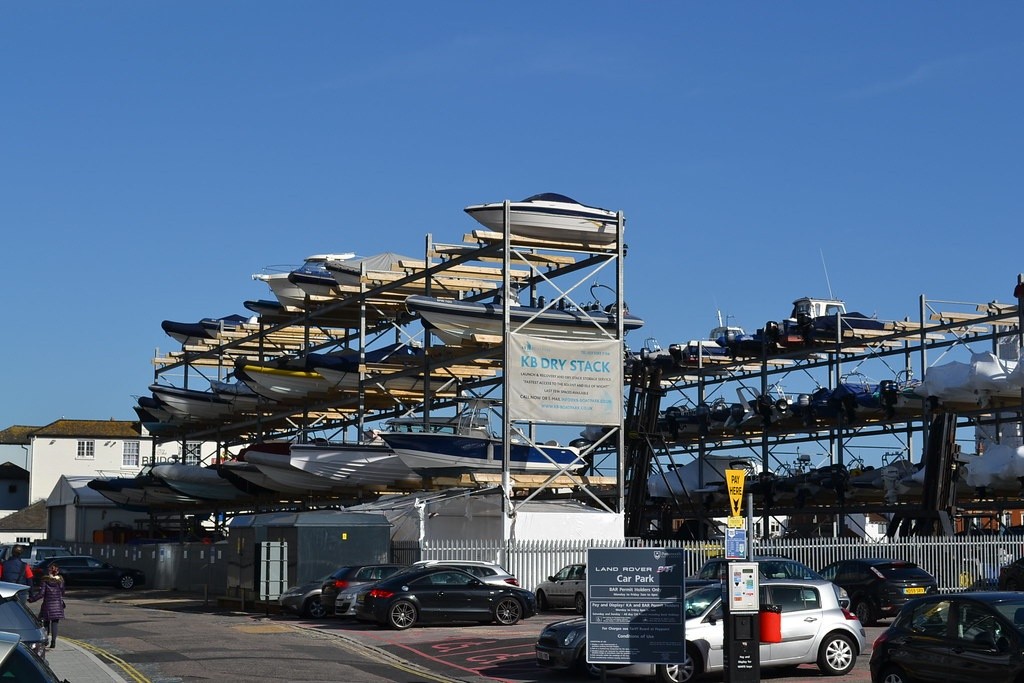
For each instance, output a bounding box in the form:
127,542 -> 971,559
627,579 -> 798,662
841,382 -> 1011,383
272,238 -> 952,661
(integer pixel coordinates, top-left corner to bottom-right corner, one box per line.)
152,200 -> 1024,515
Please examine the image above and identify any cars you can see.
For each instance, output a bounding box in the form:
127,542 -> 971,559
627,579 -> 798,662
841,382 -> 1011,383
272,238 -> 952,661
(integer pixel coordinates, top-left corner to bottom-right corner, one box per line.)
31,555 -> 146,592
587,578 -> 867,682
278,564 -> 348,620
536,563 -> 587,614
532,579 -> 717,672
1000,557 -> 1024,593
866,591 -> 1024,683
355,566 -> 537,630
0,580 -> 69,683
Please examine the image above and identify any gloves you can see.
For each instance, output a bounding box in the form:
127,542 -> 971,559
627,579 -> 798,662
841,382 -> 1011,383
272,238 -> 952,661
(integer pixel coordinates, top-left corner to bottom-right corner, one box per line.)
27,598 -> 32,603
29,592 -> 36,601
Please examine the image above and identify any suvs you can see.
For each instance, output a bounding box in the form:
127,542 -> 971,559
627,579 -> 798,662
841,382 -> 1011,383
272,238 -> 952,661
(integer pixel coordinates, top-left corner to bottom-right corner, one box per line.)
685,552 -> 852,609
321,563 -> 410,614
816,559 -> 939,626
336,559 -> 522,618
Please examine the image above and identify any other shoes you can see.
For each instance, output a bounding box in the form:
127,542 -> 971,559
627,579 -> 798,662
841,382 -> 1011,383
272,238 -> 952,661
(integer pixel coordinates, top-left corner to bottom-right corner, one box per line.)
50,641 -> 55,648
45,640 -> 49,646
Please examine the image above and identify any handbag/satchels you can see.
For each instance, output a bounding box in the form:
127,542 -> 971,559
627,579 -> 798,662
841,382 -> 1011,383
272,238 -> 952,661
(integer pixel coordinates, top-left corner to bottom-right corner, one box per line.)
62,599 -> 66,609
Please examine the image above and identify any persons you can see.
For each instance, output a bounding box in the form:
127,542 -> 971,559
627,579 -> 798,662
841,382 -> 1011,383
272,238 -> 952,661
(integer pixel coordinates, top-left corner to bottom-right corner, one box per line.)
0,544 -> 35,598
28,561 -> 65,648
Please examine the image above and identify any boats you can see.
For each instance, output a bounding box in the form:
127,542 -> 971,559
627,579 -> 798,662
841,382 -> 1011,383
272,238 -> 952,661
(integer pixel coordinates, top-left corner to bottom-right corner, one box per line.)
84,192 -> 1022,563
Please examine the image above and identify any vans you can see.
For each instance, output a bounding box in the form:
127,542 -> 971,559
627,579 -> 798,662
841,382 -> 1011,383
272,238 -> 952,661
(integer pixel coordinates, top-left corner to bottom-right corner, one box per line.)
0,542 -> 76,584
